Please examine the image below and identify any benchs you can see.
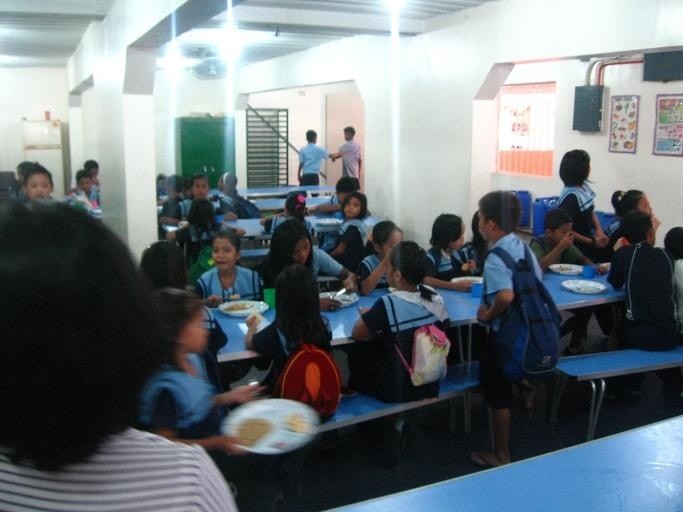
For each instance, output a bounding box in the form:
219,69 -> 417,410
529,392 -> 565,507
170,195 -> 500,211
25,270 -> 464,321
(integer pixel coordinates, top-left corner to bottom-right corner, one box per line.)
236,248 -> 271,268
548,346 -> 682,441
315,276 -> 360,292
217,361 -> 494,451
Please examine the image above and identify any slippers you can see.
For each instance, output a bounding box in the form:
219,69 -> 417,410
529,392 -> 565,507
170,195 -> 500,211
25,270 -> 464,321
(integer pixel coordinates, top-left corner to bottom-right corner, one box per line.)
476,450 -> 498,468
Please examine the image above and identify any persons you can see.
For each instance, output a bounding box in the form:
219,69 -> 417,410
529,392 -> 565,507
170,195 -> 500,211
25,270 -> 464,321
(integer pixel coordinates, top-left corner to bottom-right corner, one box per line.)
308,178 -> 361,215
20,167 -> 53,203
136,290 -> 267,461
606,210 -> 674,352
323,192 -> 369,270
556,149 -> 608,261
463,191 -> 543,466
244,266 -> 341,422
66,160 -> 101,197
351,240 -> 450,429
664,227 -> 683,345
6,162 -> 39,201
166,200 -> 245,288
329,192 -> 369,291
0,204 -> 238,512
135,240 -> 227,361
191,232 -> 267,392
423,213 -> 484,355
530,209 -> 596,354
160,174 -> 238,226
347,221 -> 404,389
208,172 -> 249,220
158,175 -> 186,239
605,189 -> 660,263
457,209 -> 486,275
333,127 -> 361,193
298,130 -> 334,197
66,170 -> 104,217
261,219 -> 358,316
261,193 -> 319,244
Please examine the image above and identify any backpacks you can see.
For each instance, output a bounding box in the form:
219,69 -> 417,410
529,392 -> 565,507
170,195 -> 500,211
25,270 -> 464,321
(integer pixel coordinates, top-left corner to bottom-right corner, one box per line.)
495,244 -> 562,396
221,198 -> 261,220
184,244 -> 218,287
393,319 -> 450,389
427,247 -> 467,274
278,320 -> 339,416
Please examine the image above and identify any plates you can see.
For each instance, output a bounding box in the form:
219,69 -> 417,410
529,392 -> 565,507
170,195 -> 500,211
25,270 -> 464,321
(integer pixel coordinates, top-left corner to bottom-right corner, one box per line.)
561,280 -> 607,294
450,277 -> 483,283
218,398 -> 321,455
318,292 -> 360,308
217,300 -> 270,316
313,219 -> 344,226
549,264 -> 584,275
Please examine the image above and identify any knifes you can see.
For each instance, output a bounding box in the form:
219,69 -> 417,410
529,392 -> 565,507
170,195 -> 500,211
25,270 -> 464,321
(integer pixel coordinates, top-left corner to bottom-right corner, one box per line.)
328,287 -> 346,302
